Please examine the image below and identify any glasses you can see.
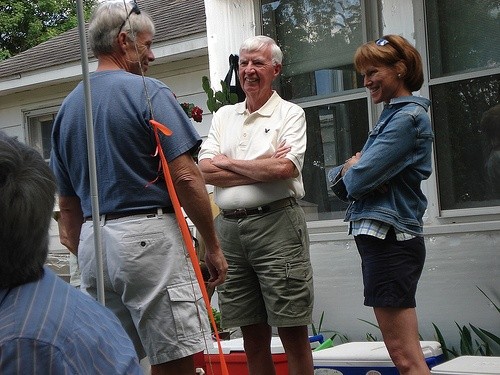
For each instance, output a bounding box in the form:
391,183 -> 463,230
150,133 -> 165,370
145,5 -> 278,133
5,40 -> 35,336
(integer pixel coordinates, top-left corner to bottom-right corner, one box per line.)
375,38 -> 403,58
117,0 -> 141,38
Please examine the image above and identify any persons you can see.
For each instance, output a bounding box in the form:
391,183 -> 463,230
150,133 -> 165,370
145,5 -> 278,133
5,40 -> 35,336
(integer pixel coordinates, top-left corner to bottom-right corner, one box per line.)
197,36 -> 319,375
329,34 -> 438,375
0,130 -> 145,375
51,0 -> 227,375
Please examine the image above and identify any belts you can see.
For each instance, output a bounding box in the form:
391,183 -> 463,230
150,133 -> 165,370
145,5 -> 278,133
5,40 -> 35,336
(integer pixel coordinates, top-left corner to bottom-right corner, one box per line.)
219,197 -> 296,219
85,206 -> 175,220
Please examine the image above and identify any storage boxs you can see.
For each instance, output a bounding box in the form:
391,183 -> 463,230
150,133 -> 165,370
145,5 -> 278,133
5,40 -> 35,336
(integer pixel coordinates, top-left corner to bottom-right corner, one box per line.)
313,341 -> 444,375
204,335 -> 320,375
